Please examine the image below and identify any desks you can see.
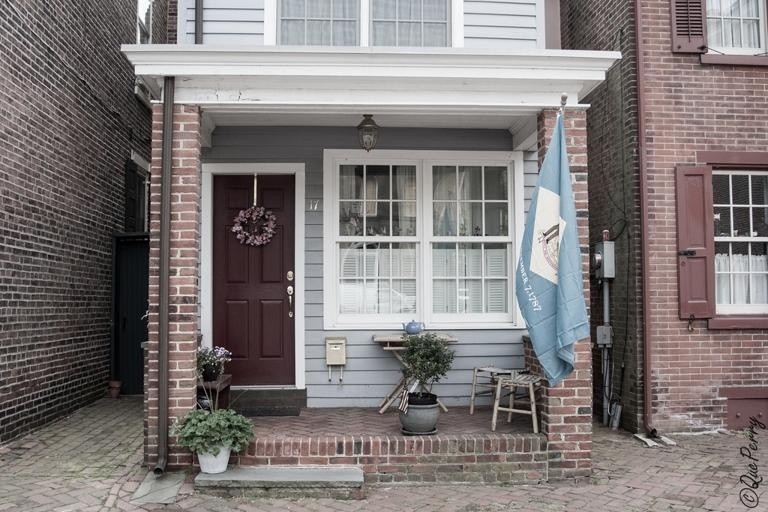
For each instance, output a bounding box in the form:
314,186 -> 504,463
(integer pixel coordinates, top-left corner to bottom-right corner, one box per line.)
371,332 -> 459,416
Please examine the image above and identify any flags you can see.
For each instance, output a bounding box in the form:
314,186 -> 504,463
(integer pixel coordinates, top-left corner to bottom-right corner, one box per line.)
512,111 -> 592,390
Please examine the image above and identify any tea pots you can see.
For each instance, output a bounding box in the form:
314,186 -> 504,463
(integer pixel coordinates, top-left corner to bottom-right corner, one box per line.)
401,319 -> 425,334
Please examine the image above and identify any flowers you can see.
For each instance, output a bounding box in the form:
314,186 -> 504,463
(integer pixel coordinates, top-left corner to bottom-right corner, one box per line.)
196,345 -> 232,375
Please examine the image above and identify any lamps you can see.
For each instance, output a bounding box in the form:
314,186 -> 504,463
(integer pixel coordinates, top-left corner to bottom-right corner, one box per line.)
356,113 -> 381,152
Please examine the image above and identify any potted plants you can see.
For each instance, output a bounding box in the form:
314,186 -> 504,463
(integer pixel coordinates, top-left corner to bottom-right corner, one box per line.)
171,408 -> 252,474
398,331 -> 456,438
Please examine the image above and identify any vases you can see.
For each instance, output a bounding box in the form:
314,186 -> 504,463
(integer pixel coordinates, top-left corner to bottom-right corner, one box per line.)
201,364 -> 220,381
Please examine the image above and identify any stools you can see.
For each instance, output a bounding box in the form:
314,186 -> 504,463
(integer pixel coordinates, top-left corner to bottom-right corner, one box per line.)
469,362 -> 542,434
